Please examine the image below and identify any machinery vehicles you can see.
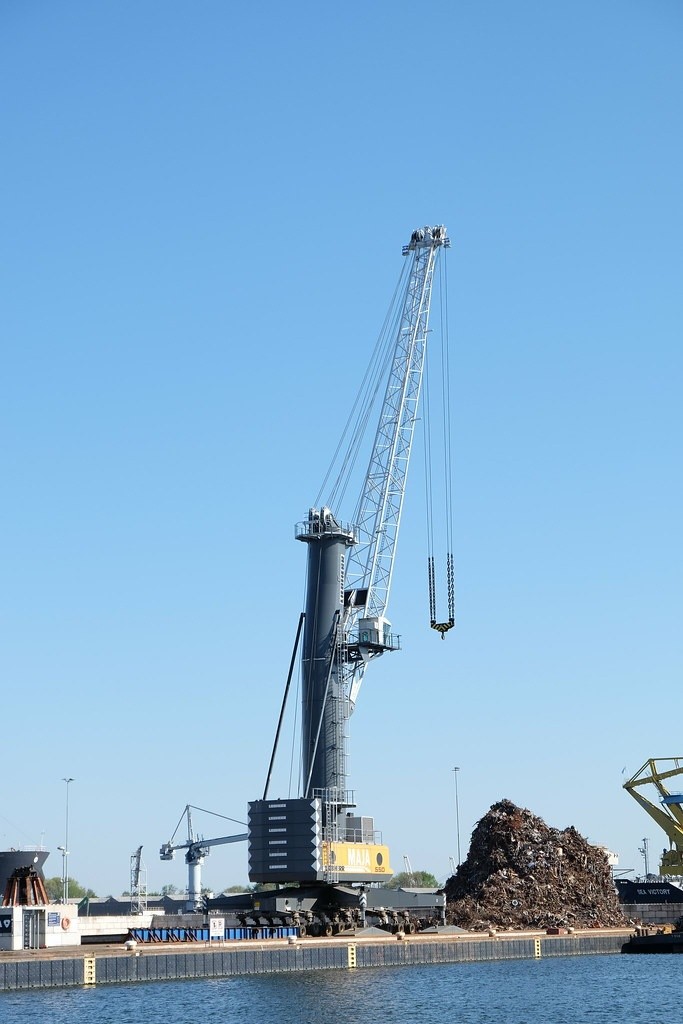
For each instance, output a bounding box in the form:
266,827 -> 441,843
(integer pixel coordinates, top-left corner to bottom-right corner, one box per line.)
199,223 -> 457,938
622,756 -> 683,877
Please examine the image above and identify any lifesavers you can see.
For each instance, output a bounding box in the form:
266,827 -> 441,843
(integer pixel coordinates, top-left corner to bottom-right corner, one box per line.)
62,917 -> 71,930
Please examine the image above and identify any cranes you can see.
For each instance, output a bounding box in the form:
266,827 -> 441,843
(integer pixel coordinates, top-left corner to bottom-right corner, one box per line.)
160,804 -> 250,860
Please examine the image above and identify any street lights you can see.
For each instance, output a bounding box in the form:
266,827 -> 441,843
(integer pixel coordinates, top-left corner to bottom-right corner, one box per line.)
58,777 -> 74,903
